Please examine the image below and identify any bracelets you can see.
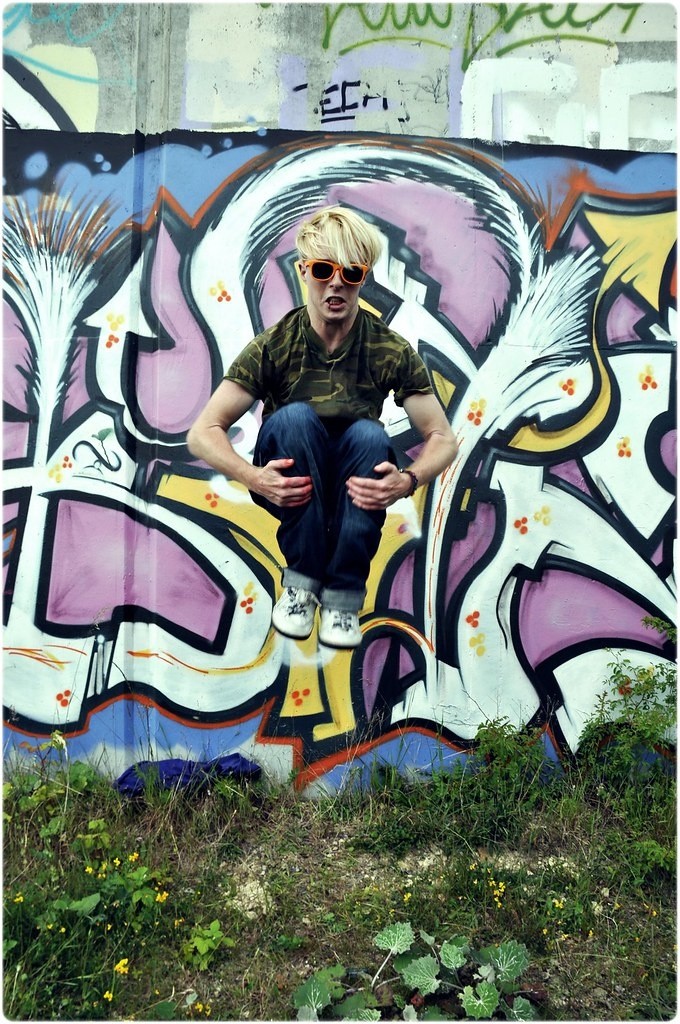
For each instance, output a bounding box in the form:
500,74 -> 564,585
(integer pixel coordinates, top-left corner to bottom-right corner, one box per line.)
399,469 -> 419,499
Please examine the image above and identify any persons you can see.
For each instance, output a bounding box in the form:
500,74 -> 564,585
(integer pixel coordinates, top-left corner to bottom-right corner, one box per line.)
185,203 -> 461,651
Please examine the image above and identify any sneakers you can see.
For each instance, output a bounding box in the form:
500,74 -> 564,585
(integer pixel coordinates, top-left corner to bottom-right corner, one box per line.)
319,607 -> 362,648
270,587 -> 321,639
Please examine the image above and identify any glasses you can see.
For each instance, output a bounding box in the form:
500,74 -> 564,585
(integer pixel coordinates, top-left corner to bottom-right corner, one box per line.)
304,260 -> 368,286
